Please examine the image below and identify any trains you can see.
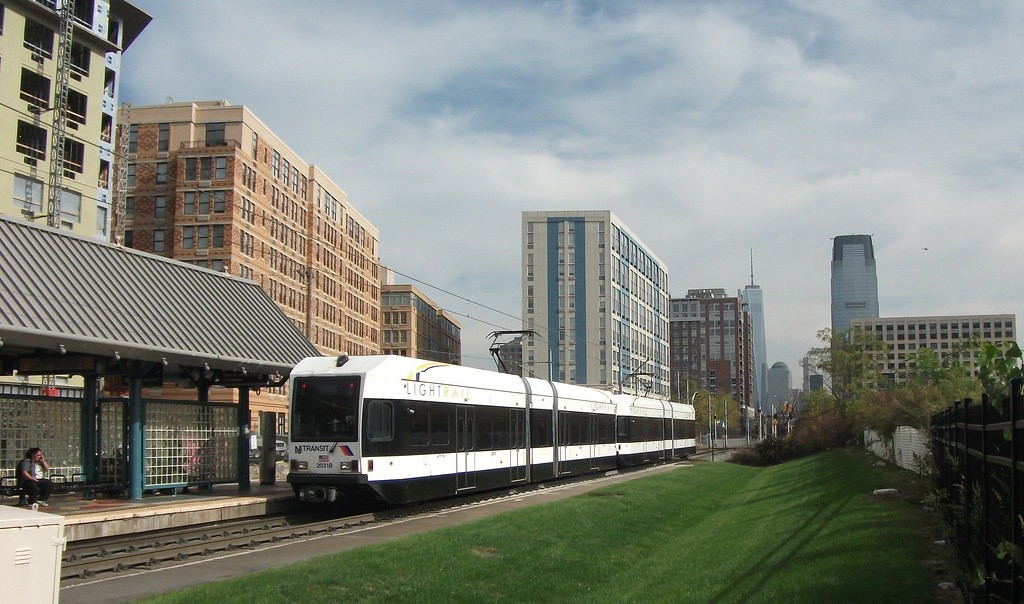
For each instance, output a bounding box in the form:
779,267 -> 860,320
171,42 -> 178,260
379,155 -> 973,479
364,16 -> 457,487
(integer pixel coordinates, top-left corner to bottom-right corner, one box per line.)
282,326 -> 698,515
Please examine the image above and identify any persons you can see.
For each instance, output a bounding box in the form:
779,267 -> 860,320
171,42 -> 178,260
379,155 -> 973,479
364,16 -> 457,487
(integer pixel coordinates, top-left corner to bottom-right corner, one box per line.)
17,448 -> 54,507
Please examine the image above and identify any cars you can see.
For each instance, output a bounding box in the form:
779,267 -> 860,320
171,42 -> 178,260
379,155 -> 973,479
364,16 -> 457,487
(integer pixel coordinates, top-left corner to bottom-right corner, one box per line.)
248,430 -> 287,464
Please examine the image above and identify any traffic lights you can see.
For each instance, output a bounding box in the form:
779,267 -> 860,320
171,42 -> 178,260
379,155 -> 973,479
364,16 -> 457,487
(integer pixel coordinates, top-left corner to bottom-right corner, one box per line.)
783,402 -> 792,412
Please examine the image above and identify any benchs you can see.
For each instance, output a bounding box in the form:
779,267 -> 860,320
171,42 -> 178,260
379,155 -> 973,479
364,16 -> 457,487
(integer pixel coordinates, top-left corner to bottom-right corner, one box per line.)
0,471 -> 127,508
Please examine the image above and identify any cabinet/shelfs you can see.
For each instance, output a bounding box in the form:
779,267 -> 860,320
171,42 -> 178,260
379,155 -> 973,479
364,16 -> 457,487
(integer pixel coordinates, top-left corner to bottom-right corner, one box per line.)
0,504 -> 67,604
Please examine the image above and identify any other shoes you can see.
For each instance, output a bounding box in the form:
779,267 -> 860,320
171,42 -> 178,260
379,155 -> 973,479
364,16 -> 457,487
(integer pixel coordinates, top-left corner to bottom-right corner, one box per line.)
37,500 -> 48,507
28,503 -> 32,507
